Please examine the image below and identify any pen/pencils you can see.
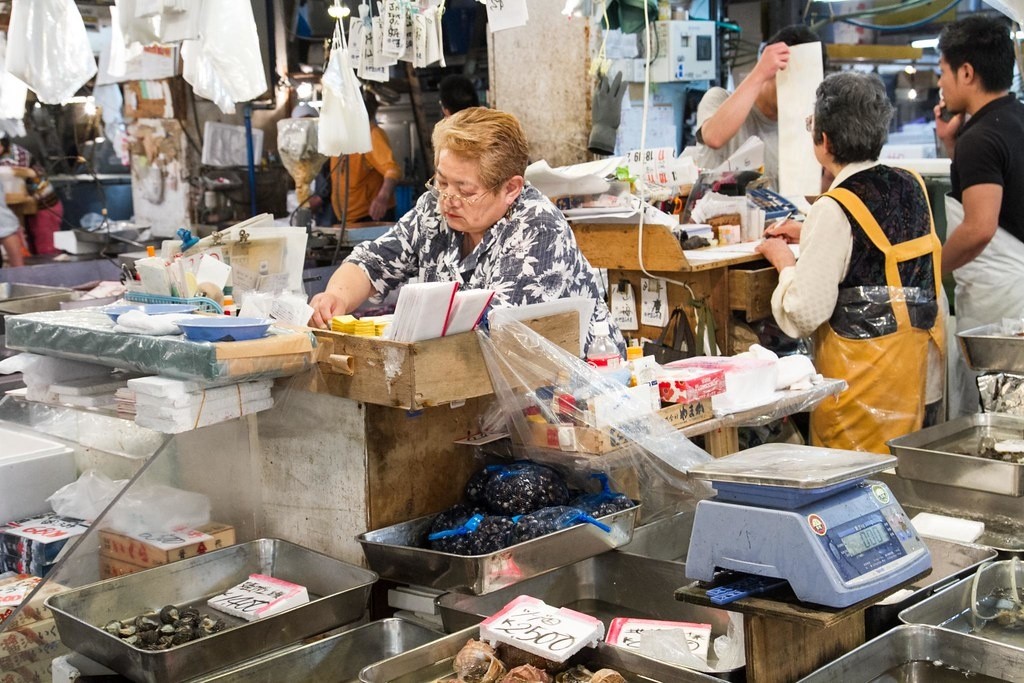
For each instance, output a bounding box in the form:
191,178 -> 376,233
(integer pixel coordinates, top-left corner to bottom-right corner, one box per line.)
668,192 -> 682,215
762,210 -> 796,242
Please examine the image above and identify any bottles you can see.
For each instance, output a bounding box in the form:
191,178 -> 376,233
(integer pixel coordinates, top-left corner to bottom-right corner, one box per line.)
587,321 -> 619,371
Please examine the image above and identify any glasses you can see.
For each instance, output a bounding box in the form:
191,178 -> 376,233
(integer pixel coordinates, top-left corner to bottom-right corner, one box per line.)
425,173 -> 502,206
805,114 -> 815,133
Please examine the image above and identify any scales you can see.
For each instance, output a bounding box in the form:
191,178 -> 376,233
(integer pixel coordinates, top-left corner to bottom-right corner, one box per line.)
684,443 -> 933,617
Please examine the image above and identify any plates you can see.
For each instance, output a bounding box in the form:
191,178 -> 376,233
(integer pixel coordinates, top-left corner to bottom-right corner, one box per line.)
171,314 -> 277,342
100,303 -> 200,323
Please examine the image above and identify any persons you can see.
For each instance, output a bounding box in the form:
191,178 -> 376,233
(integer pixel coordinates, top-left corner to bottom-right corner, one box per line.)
928,16 -> 1023,416
752,69 -> 949,468
438,74 -> 484,118
692,25 -> 837,206
328,84 -> 403,226
306,107 -> 632,426
0,129 -> 66,255
0,193 -> 28,279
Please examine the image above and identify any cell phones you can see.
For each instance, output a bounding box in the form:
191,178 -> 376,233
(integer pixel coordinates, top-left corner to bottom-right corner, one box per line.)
939,104 -> 960,123
